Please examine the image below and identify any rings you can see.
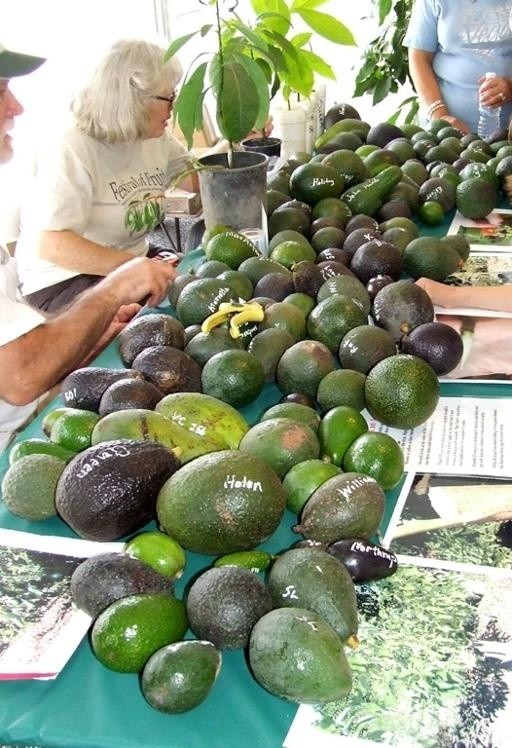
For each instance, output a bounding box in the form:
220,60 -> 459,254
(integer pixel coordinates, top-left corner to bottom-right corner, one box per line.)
497,92 -> 507,100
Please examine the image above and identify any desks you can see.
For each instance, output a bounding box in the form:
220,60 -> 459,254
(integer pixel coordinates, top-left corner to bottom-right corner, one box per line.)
1,199 -> 511,748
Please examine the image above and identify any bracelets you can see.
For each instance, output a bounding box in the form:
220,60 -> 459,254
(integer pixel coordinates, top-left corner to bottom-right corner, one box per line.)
427,99 -> 446,118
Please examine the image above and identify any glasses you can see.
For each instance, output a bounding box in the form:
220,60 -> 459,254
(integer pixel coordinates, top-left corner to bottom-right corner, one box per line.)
150,88 -> 178,102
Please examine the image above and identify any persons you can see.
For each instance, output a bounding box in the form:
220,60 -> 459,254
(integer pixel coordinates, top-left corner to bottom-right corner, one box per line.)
401,1 -> 511,142
15,35 -> 274,321
0,39 -> 176,463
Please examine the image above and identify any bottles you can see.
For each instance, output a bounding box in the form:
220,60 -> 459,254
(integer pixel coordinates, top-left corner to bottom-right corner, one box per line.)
478,71 -> 500,141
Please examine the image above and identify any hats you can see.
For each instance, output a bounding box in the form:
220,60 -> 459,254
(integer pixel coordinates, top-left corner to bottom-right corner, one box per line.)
0,42 -> 49,80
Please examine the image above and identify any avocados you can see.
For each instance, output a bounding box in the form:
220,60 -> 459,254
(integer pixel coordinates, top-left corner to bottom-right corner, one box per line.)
249,608 -> 354,705
267,547 -> 362,649
2,104 -> 512,582
70,554 -> 173,617
186,566 -> 272,650
140,638 -> 223,715
90,593 -> 190,674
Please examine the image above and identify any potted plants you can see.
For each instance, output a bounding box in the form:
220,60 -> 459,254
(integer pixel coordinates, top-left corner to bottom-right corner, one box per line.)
162,1 -> 290,243
222,1 -> 357,158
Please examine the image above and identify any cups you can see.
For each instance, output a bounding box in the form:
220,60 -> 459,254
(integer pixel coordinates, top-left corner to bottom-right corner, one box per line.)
238,227 -> 267,258
